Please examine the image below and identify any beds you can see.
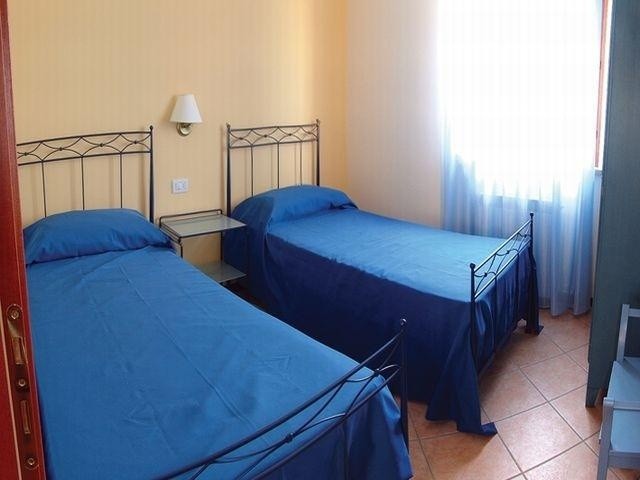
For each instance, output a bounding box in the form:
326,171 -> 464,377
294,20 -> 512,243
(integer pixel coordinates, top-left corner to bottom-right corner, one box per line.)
221,121 -> 543,436
11,120 -> 421,480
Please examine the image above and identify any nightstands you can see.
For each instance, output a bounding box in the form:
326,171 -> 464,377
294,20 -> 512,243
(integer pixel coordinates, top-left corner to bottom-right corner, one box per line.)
158,207 -> 253,305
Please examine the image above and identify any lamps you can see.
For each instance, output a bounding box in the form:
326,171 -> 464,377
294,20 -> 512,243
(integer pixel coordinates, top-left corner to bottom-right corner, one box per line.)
170,93 -> 204,137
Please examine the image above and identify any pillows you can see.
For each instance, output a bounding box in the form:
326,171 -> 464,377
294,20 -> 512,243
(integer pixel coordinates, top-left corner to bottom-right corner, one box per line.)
23,207 -> 176,271
230,182 -> 360,235
